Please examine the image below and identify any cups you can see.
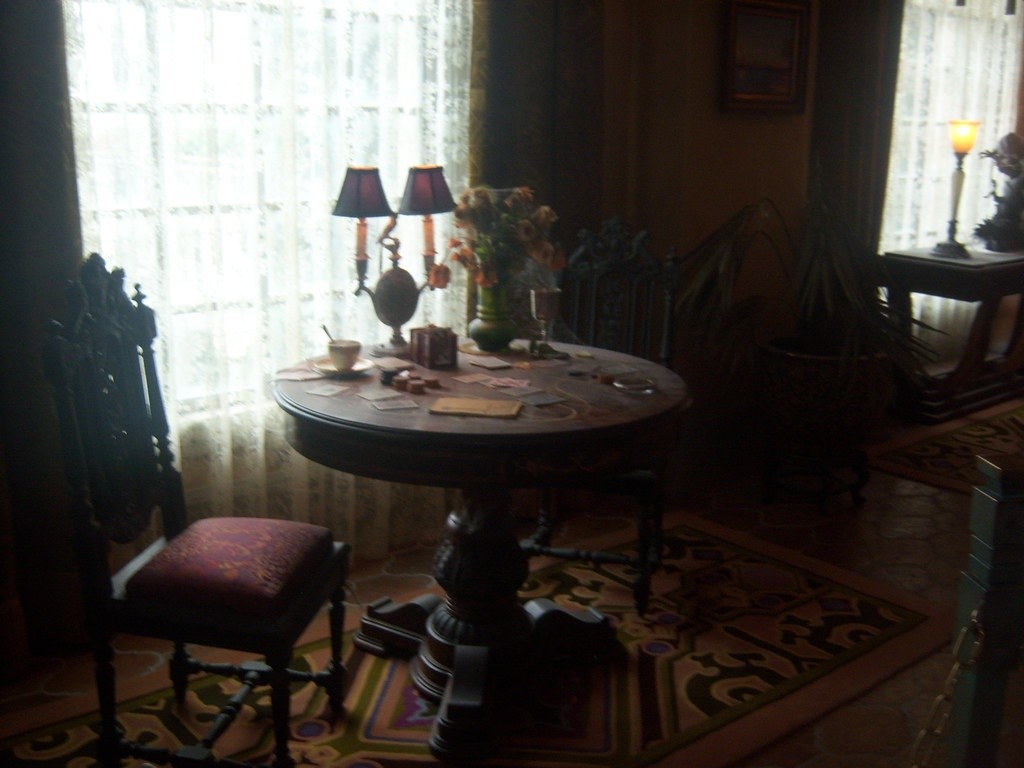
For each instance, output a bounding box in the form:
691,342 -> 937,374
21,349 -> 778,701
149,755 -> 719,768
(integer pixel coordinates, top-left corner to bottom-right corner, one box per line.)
328,340 -> 361,371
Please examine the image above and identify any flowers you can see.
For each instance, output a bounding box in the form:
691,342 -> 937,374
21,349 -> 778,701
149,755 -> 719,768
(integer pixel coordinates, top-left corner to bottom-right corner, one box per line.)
448,185 -> 557,286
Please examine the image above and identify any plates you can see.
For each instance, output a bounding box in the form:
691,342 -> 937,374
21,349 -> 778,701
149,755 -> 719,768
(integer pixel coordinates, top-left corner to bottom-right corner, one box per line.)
313,358 -> 374,373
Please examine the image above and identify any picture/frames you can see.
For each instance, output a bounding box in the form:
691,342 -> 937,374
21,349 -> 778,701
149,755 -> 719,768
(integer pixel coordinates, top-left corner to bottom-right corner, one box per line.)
717,0 -> 809,114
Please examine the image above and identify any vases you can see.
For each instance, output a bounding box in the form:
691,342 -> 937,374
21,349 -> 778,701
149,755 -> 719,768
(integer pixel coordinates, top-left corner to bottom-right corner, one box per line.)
466,286 -> 519,354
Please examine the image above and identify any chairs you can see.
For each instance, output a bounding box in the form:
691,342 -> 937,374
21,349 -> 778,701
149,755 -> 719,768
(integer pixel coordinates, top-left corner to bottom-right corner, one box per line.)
40,252 -> 355,768
515,214 -> 684,616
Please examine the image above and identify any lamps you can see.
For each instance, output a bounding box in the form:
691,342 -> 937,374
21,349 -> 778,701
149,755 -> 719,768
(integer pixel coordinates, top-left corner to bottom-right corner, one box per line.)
929,120 -> 983,259
332,164 -> 460,359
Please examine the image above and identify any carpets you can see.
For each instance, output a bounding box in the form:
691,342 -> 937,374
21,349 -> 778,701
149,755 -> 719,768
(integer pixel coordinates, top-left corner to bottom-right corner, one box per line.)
844,397 -> 1024,496
1,511 -> 962,768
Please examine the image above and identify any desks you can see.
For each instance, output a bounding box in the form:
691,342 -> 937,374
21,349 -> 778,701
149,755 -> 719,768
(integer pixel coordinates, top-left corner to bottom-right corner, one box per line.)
269,335 -> 696,768
878,250 -> 1024,426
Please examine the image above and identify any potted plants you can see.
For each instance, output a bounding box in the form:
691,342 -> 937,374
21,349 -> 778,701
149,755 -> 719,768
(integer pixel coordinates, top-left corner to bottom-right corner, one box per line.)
670,188 -> 952,456
